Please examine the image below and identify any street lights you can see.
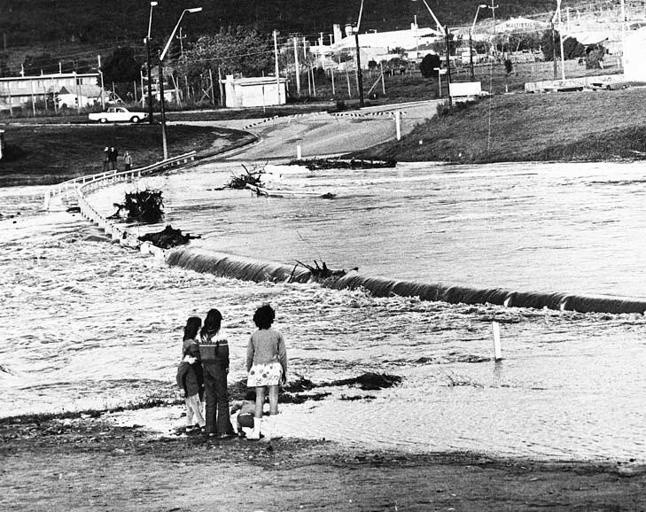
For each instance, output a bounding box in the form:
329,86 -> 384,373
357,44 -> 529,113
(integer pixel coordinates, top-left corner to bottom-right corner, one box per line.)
158,8 -> 203,161
147,2 -> 158,122
469,5 -> 487,80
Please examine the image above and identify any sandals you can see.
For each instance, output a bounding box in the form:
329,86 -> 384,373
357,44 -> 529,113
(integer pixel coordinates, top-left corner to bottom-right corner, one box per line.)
185,423 -> 236,439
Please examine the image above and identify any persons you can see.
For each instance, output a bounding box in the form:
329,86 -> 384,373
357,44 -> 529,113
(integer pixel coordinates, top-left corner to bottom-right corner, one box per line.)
101,145 -> 111,173
108,146 -> 119,171
243,305 -> 288,443
181,316 -> 207,432
122,150 -> 133,176
174,339 -> 207,436
193,308 -> 238,441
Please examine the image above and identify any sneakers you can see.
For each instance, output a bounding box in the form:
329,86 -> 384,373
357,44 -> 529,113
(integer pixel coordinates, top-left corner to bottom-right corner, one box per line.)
246,431 -> 261,440
271,432 -> 283,439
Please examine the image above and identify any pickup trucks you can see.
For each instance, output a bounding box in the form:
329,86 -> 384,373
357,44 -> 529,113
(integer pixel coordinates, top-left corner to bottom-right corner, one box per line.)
88,106 -> 149,123
